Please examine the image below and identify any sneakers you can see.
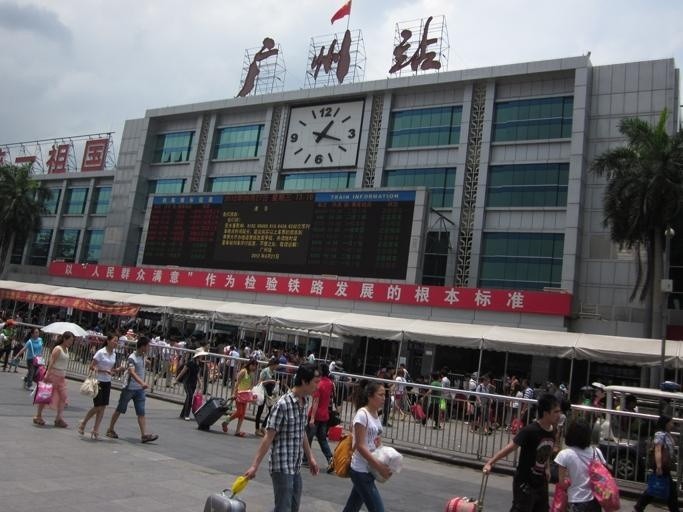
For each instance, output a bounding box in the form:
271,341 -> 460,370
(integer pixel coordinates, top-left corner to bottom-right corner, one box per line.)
106,429 -> 118,438
142,434 -> 158,442
33,416 -> 67,427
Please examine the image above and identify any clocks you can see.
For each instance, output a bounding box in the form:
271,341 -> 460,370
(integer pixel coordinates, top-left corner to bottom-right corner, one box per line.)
279,97 -> 366,172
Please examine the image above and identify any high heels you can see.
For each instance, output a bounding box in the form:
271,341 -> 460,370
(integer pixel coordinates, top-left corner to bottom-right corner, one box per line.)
78,425 -> 99,440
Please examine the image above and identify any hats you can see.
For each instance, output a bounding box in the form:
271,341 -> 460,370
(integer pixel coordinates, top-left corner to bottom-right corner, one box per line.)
192,347 -> 208,358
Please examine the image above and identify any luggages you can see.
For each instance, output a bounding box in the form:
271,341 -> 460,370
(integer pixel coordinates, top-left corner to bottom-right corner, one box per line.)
204,489 -> 246,512
445,470 -> 488,512
404,393 -> 425,420
194,397 -> 235,426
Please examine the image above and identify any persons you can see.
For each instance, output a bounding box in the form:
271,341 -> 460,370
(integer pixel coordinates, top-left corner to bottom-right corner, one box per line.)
342,383 -> 393,512
245,364 -> 320,512
13,328 -> 43,391
33,331 -> 75,427
579,387 -> 622,463
483,394 -> 562,512
377,363 -> 451,430
254,357 -> 280,437
634,414 -> 680,512
107,337 -> 158,443
222,359 -> 258,436
172,347 -> 209,420
7,341 -> 24,373
220,338 -> 344,407
77,333 -> 123,439
460,372 -> 569,435
302,363 -> 337,474
554,418 -> 606,512
113,328 -> 187,392
1,319 -> 16,372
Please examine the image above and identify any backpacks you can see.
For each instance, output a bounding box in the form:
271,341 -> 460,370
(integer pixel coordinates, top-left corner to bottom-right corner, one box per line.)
331,409 -> 368,478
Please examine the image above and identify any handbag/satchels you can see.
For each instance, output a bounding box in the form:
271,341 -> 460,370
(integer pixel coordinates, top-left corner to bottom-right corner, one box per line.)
33,356 -> 46,367
577,448 -> 620,512
647,474 -> 670,499
80,378 -> 99,398
329,411 -> 340,427
33,381 -> 54,404
236,390 -> 253,403
551,480 -> 570,512
